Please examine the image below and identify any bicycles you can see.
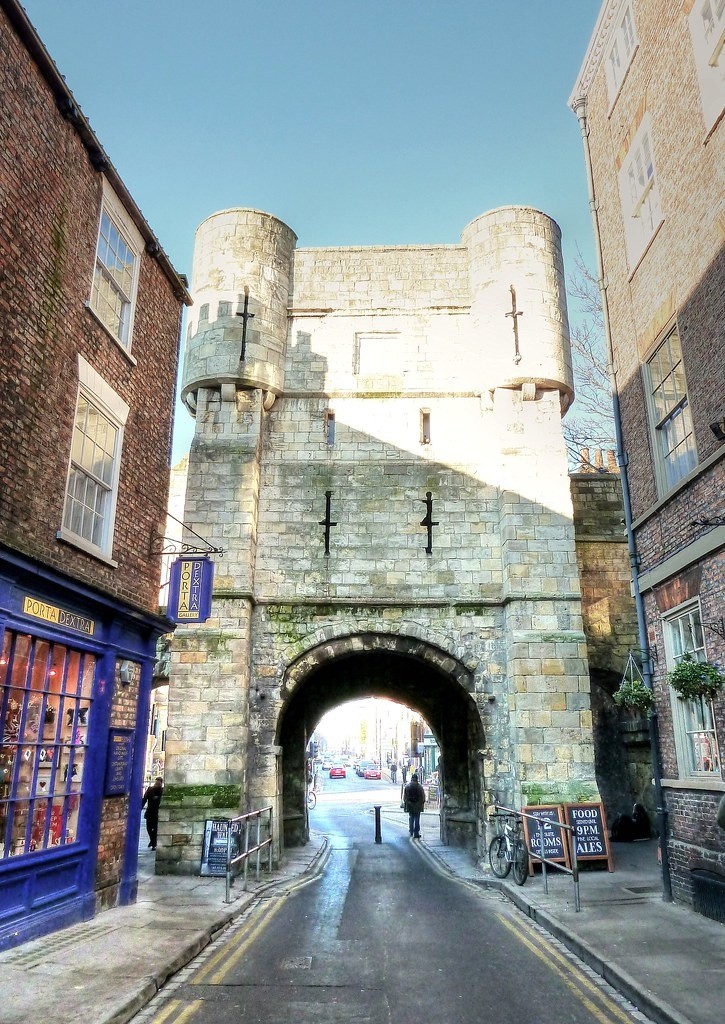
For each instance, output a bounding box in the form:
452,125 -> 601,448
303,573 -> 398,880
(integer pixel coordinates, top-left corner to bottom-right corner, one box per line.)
486,811 -> 529,885
307,791 -> 316,810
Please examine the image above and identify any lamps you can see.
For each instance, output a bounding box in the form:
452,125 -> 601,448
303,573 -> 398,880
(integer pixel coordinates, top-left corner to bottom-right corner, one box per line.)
709,416 -> 725,441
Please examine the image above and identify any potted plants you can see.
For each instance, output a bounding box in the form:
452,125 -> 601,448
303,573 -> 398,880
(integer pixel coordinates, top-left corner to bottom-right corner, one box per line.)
612,679 -> 657,722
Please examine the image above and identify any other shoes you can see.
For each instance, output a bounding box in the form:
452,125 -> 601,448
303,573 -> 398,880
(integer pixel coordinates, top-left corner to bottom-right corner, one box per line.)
415,835 -> 421,838
411,833 -> 413,835
148,841 -> 152,847
152,847 -> 156,850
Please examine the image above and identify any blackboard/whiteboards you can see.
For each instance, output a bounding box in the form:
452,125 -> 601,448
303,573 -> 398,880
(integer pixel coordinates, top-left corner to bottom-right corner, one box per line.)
563,802 -> 612,860
199,819 -> 242,877
521,805 -> 569,863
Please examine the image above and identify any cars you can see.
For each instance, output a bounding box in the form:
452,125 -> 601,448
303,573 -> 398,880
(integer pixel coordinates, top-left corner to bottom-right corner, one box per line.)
363,764 -> 383,780
322,757 -> 375,776
328,764 -> 346,778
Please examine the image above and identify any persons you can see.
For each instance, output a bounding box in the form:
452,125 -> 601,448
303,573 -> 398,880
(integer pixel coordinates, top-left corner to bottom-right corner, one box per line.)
391,762 -> 397,783
403,774 -> 425,838
141,777 -> 163,851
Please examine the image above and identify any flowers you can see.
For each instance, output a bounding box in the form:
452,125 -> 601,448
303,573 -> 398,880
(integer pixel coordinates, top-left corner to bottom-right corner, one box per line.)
666,652 -> 724,705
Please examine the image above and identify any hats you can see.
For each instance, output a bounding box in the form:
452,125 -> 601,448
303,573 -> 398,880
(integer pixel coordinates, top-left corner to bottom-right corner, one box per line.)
412,774 -> 418,778
156,777 -> 163,782
